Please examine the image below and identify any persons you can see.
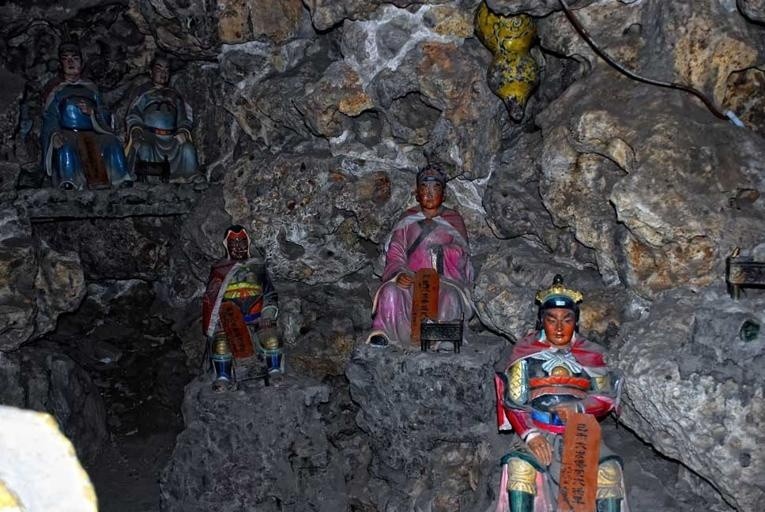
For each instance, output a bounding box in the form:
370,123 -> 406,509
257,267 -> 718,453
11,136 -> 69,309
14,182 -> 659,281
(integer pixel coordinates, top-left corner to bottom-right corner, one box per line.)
204,224 -> 285,392
42,42 -> 134,190
494,273 -> 626,512
123,53 -> 199,185
366,165 -> 474,350
